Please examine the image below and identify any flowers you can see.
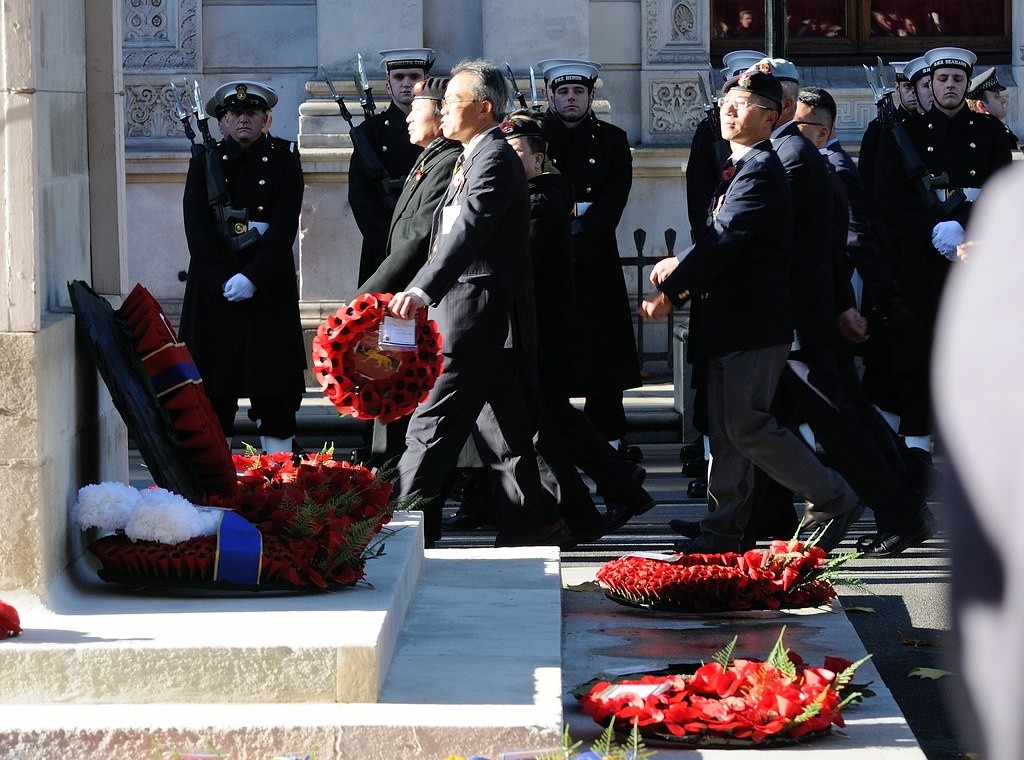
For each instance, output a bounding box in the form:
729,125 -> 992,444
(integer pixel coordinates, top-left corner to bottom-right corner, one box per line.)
502,121 -> 517,134
63,279 -> 897,748
738,72 -> 751,89
722,167 -> 736,179
415,160 -> 425,180
756,63 -> 772,75
412,81 -> 425,92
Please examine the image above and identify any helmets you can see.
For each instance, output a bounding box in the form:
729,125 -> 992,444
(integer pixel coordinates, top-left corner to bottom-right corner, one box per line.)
537,58 -> 601,93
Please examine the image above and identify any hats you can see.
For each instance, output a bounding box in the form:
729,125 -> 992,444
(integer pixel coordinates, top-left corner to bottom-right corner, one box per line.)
888,62 -> 911,82
902,56 -> 930,85
924,46 -> 977,79
500,117 -> 543,141
720,49 -> 769,81
411,77 -> 452,100
744,57 -> 799,85
378,48 -> 436,78
966,67 -> 1006,99
722,64 -> 784,102
205,80 -> 279,123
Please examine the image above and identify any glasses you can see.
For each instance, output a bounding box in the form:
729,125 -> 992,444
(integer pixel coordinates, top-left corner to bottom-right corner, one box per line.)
718,97 -> 774,111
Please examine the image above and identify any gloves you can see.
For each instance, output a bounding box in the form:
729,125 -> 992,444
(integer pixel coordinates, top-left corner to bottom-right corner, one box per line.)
223,272 -> 257,302
931,220 -> 966,261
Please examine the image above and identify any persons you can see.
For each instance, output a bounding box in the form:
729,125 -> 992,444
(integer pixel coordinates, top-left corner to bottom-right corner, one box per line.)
712,10 -> 936,42
182,48 -> 1024,564
931,160 -> 1024,760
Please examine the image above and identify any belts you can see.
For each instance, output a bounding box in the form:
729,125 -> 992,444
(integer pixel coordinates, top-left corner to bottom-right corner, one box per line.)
232,219 -> 269,237
570,202 -> 594,217
934,187 -> 982,203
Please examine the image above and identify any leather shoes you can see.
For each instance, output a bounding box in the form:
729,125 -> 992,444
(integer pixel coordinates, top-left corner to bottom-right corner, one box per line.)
855,509 -> 939,559
441,503 -> 506,531
687,478 -> 706,495
681,456 -> 708,477
673,502 -> 866,554
680,434 -> 702,461
924,456 -> 937,496
668,518 -> 755,540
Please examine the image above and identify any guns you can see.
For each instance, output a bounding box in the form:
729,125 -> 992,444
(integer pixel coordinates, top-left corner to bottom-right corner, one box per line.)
163,79 -> 262,250
319,54 -> 411,203
502,63 -> 580,197
859,55 -> 972,228
696,62 -> 737,187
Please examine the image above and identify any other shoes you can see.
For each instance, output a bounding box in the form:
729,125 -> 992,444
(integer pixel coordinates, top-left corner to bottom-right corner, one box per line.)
607,437 -> 644,461
495,517 -> 577,549
602,485 -> 657,532
589,464 -> 646,505
564,499 -> 608,544
